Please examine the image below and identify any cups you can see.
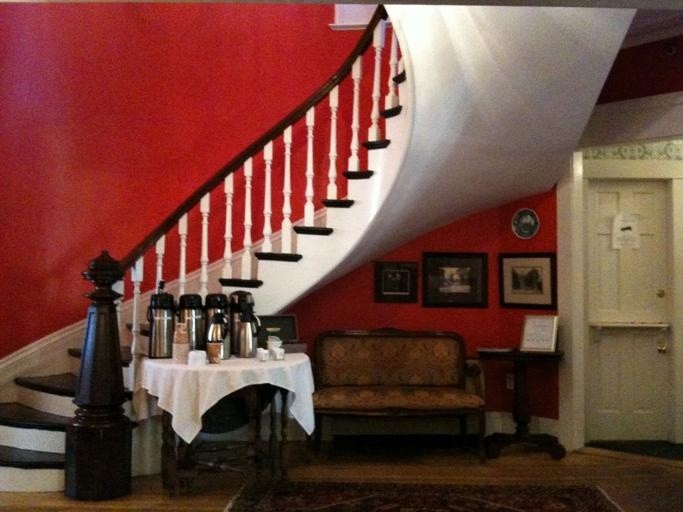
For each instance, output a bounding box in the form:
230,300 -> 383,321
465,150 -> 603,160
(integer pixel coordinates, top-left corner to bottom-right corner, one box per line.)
206,340 -> 224,365
253,338 -> 287,363
187,349 -> 207,367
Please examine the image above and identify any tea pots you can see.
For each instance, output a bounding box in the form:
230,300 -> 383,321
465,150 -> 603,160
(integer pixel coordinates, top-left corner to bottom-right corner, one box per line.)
207,312 -> 232,361
235,309 -> 261,359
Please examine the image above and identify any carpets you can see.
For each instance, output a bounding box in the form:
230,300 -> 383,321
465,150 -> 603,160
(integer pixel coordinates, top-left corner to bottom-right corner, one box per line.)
224,476 -> 626,512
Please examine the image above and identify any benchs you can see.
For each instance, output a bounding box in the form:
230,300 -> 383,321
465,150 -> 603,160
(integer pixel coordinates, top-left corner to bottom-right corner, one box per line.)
470,340 -> 567,465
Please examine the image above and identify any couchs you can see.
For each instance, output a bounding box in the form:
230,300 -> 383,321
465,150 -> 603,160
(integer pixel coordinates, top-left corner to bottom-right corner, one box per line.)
305,329 -> 488,459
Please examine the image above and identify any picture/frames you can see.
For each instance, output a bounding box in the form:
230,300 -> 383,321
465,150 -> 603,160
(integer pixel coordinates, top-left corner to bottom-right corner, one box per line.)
373,251 -> 560,352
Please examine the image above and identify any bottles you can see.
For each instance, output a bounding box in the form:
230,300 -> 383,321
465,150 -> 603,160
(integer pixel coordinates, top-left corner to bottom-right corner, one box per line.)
171,320 -> 190,365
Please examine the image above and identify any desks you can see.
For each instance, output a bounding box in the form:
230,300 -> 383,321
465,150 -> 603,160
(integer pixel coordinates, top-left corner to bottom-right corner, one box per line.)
135,348 -> 311,497
